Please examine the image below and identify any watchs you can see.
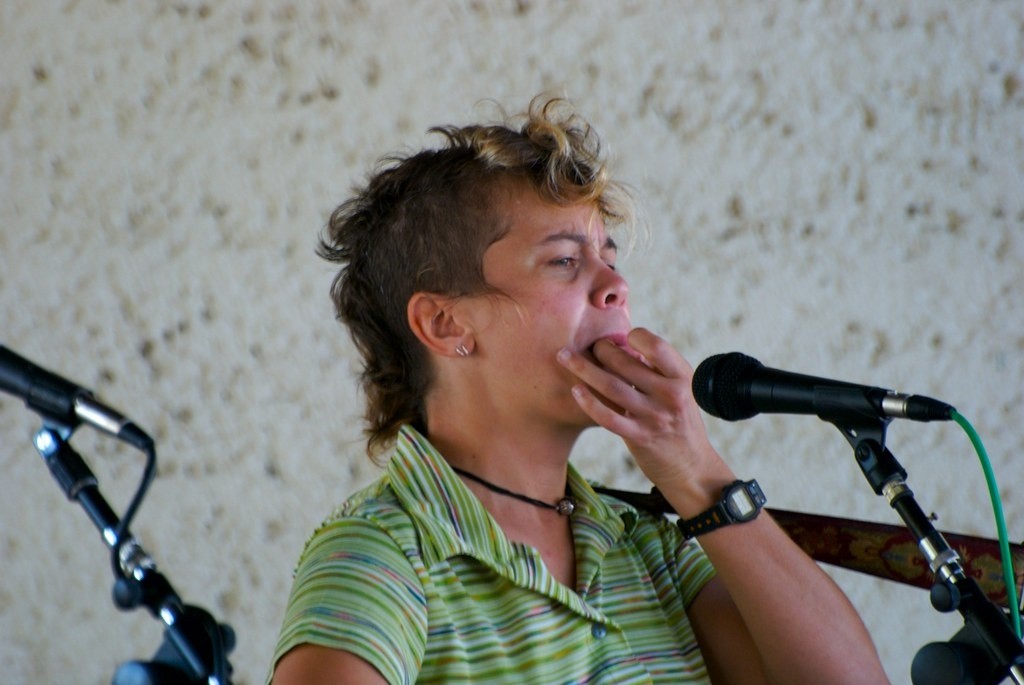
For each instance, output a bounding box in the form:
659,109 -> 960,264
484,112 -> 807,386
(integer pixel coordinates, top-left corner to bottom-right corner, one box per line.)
678,479 -> 765,538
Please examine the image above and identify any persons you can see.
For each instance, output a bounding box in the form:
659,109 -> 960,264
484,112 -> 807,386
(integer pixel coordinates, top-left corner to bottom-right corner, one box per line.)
272,91 -> 886,685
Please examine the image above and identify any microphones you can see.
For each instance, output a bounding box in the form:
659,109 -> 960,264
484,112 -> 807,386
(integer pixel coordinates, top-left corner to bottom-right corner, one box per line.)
0,346 -> 155,451
692,352 -> 956,423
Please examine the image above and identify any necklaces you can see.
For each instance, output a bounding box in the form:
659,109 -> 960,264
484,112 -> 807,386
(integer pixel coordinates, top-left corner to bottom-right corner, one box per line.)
441,467 -> 573,514
